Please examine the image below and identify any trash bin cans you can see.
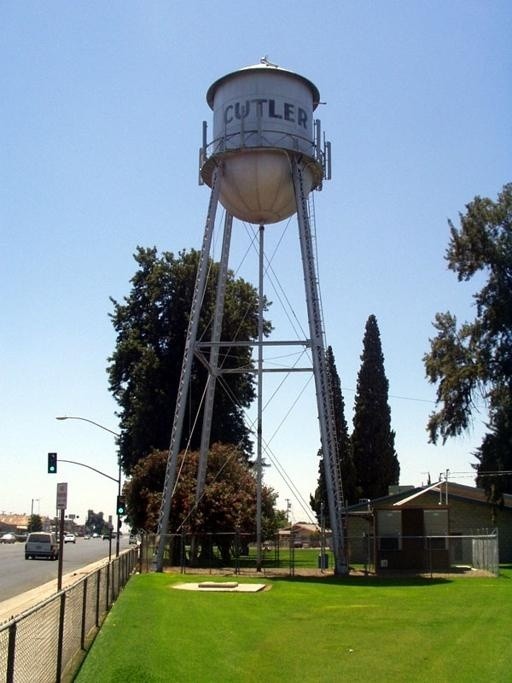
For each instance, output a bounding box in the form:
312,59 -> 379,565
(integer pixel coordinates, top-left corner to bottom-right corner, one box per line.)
318,554 -> 328,568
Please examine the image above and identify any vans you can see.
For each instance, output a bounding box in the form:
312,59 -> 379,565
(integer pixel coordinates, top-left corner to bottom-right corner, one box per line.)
128,528 -> 137,544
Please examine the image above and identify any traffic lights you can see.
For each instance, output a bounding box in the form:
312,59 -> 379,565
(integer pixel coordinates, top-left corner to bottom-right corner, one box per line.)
48,452 -> 58,473
117,495 -> 127,515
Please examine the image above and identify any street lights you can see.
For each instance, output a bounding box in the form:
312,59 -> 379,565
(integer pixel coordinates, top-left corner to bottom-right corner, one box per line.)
56,416 -> 123,562
31,498 -> 40,515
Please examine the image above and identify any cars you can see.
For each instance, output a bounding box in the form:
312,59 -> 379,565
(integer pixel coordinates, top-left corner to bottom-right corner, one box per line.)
294,540 -> 303,547
0,530 -> 122,544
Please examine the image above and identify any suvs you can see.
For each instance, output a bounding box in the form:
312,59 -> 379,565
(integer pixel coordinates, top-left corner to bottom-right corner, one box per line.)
24,531 -> 60,561
